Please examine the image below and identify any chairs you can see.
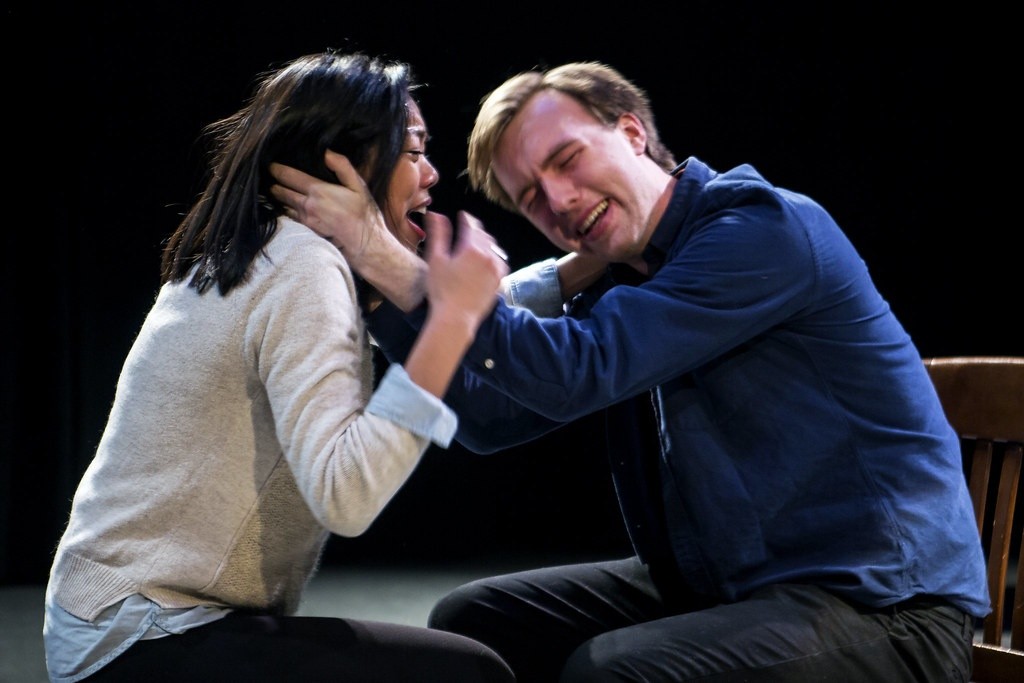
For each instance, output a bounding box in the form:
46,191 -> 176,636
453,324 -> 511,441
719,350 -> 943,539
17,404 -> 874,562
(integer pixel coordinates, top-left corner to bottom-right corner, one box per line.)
920,352 -> 1024,683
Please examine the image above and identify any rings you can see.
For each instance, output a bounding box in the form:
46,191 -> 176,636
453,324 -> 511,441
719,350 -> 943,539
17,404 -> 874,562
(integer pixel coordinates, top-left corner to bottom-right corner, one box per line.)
491,245 -> 509,261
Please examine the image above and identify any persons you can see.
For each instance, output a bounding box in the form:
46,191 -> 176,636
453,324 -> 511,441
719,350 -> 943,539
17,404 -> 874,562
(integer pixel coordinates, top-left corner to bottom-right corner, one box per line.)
44,52 -> 613,683
269,59 -> 994,683
